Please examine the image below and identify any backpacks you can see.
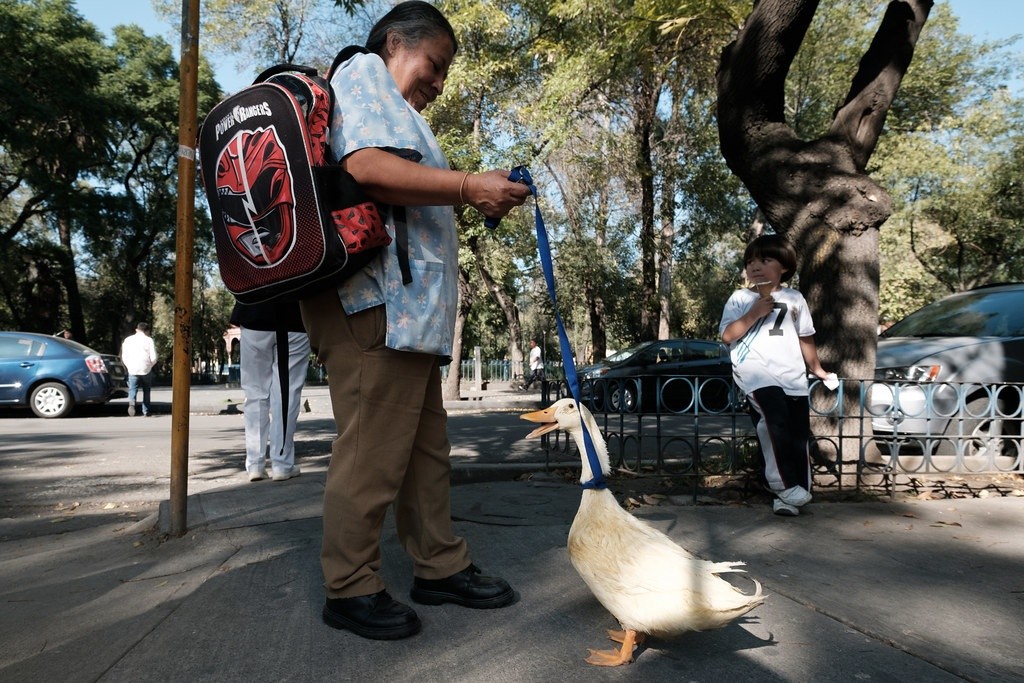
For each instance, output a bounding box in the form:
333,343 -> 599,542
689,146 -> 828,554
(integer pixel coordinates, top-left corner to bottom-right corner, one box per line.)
199,62 -> 393,310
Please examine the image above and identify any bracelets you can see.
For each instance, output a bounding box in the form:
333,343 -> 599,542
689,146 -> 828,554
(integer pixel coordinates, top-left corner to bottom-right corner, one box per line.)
459,170 -> 470,209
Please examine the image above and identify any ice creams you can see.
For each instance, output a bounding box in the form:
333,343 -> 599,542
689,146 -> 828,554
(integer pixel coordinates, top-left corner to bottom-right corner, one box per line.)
757,281 -> 773,298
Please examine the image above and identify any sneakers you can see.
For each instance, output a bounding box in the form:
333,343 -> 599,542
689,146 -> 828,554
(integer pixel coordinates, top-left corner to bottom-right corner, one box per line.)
323,590 -> 420,641
248,466 -> 301,481
411,564 -> 514,610
772,486 -> 815,516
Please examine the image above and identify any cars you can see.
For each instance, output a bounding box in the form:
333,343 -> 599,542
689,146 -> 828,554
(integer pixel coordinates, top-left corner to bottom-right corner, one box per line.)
0,331 -> 130,418
871,283 -> 1024,458
574,337 -> 749,412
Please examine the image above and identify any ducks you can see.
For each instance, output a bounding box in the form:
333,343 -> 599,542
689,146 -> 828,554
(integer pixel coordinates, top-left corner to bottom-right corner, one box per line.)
520,398 -> 772,666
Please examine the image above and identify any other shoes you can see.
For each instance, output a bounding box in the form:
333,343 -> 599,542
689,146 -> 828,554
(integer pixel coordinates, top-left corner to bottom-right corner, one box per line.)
128,410 -> 153,417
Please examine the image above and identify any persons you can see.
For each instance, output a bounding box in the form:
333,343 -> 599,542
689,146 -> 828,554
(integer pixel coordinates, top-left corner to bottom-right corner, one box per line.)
63,329 -> 73,339
299,0 -> 534,642
120,322 -> 157,416
719,234 -> 833,517
517,340 -> 547,392
229,292 -> 312,481
